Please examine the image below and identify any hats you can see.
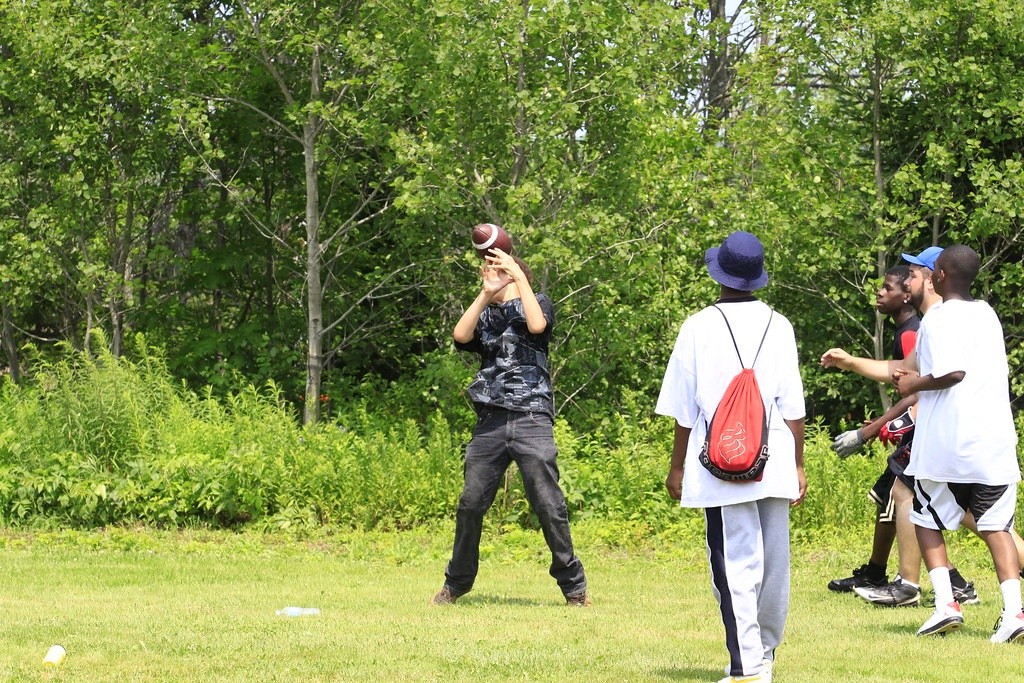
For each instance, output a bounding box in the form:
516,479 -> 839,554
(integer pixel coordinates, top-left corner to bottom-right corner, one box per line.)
705,231 -> 768,291
901,246 -> 944,271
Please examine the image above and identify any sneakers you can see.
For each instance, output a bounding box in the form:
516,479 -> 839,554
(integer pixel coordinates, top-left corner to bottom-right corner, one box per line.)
720,665 -> 772,682
828,573 -> 888,592
924,582 -> 978,606
566,594 -> 585,607
915,602 -> 964,637
433,586 -> 456,605
852,581 -> 922,608
990,608 -> 1024,643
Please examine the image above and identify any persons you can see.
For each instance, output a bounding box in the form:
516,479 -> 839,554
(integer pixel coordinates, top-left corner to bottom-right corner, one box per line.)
892,247 -> 1024,645
654,234 -> 809,683
433,246 -> 589,607
819,246 -> 1024,613
829,266 -> 981,607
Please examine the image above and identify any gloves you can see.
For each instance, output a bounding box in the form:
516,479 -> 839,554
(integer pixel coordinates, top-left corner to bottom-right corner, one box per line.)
829,428 -> 866,458
879,406 -> 914,447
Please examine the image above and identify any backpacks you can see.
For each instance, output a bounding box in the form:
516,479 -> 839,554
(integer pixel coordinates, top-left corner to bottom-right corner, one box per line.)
699,304 -> 773,483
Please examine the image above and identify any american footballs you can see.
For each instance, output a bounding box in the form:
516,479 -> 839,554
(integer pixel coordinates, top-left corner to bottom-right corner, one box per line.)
471,222 -> 513,262
